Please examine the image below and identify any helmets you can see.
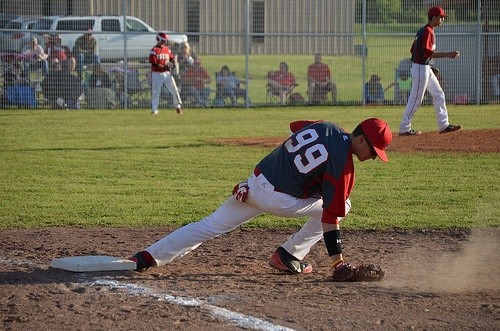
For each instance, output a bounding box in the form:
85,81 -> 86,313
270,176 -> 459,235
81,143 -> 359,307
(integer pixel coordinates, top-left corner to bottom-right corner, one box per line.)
157,33 -> 168,42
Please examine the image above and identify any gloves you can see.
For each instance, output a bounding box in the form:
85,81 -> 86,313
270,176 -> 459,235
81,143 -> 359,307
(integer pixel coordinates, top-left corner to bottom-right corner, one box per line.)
232,181 -> 249,203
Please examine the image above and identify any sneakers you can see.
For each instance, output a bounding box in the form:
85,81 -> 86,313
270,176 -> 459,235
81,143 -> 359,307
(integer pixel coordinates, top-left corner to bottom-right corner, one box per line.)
128,251 -> 157,272
268,246 -> 313,275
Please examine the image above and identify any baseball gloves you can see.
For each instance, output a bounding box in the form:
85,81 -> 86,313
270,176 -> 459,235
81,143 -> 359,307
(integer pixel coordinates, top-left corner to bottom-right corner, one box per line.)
331,265 -> 384,283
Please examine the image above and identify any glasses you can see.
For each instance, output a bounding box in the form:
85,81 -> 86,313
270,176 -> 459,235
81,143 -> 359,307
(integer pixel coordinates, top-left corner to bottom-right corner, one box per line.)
85,33 -> 92,36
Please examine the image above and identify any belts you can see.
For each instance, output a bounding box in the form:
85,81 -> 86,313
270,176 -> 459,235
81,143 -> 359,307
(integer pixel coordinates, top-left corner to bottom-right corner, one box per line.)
254,166 -> 283,192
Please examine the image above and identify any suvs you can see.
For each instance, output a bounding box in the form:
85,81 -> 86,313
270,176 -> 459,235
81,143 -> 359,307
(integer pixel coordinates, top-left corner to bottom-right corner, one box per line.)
0,14 -> 60,54
31,14 -> 190,61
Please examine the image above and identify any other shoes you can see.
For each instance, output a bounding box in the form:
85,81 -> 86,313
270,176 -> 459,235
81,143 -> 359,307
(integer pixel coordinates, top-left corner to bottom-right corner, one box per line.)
439,124 -> 463,134
399,129 -> 422,136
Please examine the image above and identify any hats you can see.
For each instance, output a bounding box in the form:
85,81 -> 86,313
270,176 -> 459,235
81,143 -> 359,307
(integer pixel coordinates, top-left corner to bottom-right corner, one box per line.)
428,6 -> 448,16
361,118 -> 392,163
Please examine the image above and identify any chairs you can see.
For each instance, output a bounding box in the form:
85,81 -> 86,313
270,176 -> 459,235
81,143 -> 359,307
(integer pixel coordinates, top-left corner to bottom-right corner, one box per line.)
308,90 -> 328,103
266,71 -> 287,104
127,68 -> 149,102
216,71 -> 238,103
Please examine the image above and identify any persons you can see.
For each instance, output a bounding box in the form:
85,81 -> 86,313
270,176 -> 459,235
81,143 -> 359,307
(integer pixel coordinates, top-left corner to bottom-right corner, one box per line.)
149,33 -> 181,114
307,53 -> 338,105
127,117 -> 393,282
365,75 -> 384,104
385,72 -> 413,104
215,65 -> 251,106
0,27 -> 119,109
174,43 -> 212,106
399,6 -> 463,135
267,62 -> 296,105
